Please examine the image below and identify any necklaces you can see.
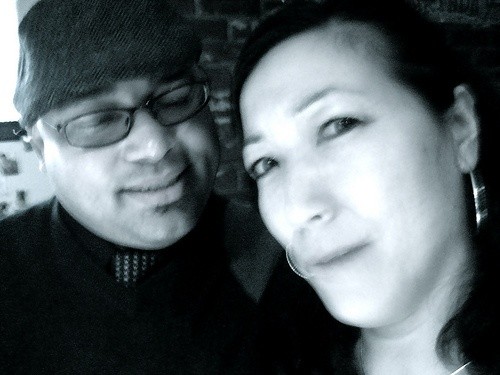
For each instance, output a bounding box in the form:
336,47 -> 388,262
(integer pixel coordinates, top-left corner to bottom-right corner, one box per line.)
449,360 -> 475,375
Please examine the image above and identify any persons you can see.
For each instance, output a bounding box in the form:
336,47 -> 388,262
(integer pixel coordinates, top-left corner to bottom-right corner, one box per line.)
229,1 -> 500,375
1,0 -> 353,375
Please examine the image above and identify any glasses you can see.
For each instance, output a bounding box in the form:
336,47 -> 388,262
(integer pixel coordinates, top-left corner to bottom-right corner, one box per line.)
42,64 -> 212,147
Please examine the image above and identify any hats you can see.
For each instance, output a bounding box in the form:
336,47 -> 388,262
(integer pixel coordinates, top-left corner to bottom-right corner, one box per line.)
14,0 -> 204,128
